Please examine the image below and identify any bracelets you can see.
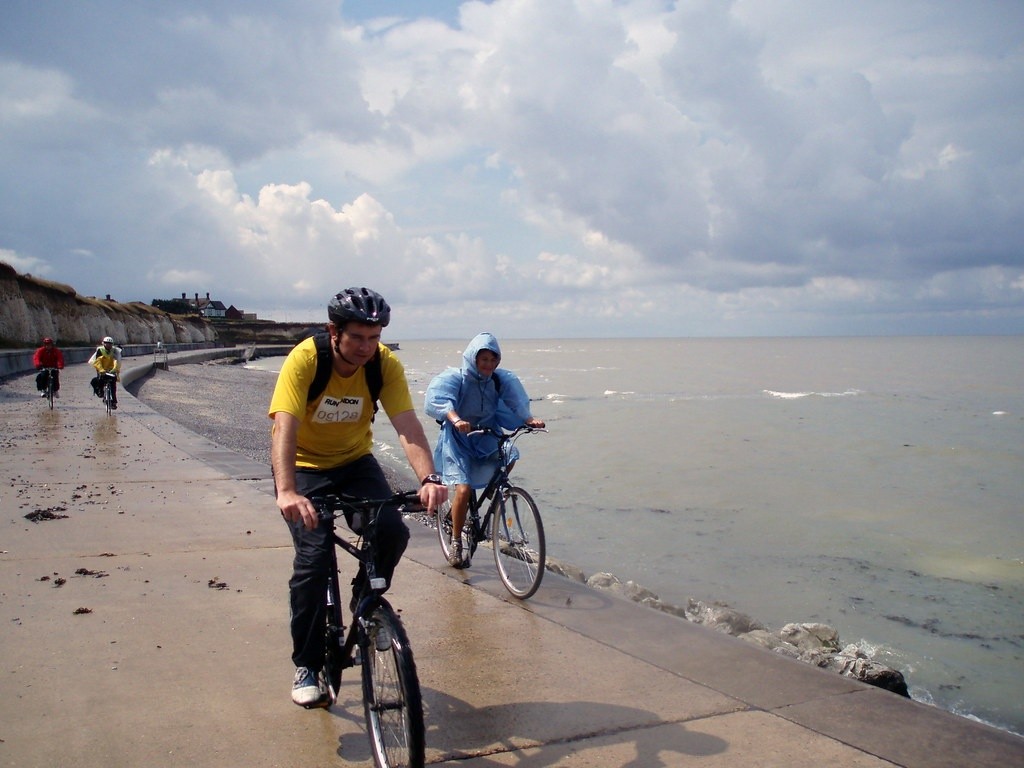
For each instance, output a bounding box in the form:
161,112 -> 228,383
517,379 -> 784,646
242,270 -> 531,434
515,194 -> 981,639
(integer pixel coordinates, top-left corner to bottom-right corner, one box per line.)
452,416 -> 461,425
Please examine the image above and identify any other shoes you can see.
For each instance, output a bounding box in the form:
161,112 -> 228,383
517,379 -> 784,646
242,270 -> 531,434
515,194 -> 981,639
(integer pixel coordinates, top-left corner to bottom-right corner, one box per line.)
111,402 -> 117,410
98,390 -> 104,397
41,389 -> 47,397
53,391 -> 59,399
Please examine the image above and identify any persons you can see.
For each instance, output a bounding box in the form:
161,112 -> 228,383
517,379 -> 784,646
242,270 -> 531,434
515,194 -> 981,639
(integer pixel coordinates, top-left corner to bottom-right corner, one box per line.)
35,337 -> 63,398
157,340 -> 163,352
89,337 -> 123,409
424,332 -> 544,566
269,287 -> 448,705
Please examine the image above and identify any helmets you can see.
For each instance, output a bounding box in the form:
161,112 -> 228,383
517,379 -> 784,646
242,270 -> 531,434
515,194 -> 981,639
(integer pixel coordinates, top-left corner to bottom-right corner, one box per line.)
103,336 -> 113,343
43,338 -> 52,343
326,286 -> 390,326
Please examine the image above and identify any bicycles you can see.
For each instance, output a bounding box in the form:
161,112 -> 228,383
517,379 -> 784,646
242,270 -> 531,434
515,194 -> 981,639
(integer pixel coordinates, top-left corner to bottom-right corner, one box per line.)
277,487 -> 439,768
91,365 -> 118,417
434,422 -> 550,599
38,367 -> 61,410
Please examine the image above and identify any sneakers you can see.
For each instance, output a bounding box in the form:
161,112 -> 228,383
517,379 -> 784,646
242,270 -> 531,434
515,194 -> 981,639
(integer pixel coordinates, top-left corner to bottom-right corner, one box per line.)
291,665 -> 320,705
447,540 -> 463,567
349,597 -> 390,650
488,487 -> 505,514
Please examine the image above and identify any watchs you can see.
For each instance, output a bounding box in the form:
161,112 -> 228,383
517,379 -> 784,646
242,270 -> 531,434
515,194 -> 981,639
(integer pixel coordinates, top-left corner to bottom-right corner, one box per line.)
422,474 -> 442,486
